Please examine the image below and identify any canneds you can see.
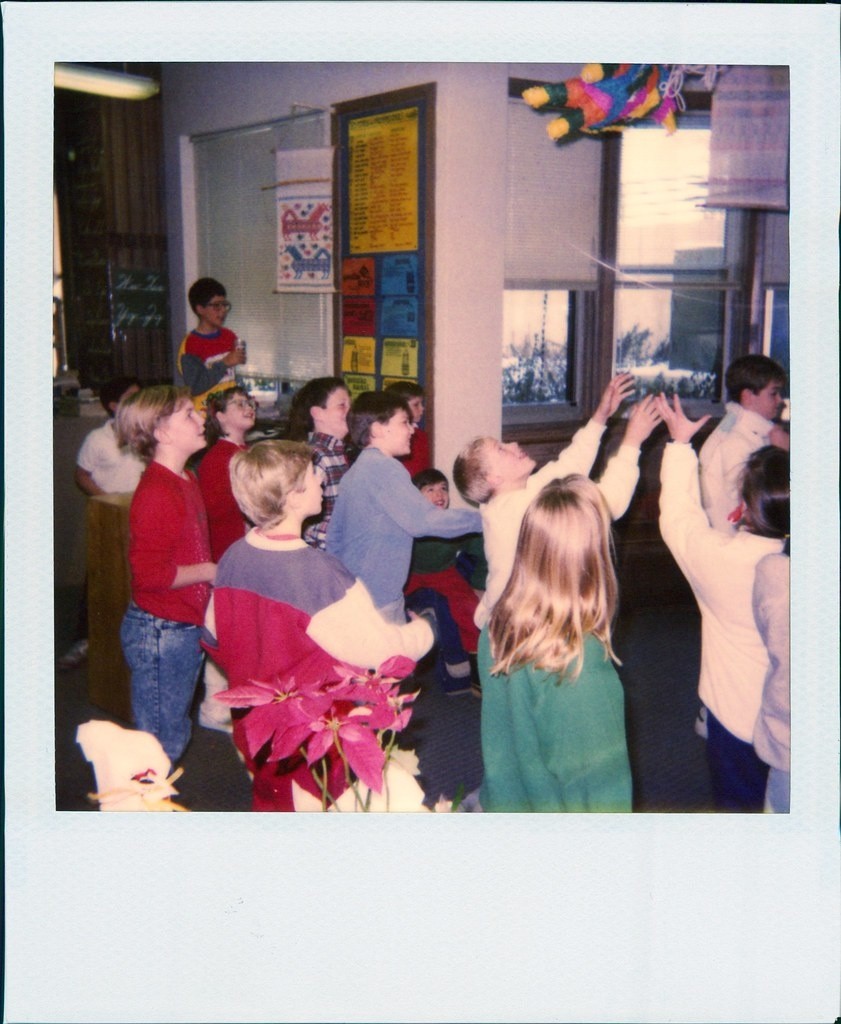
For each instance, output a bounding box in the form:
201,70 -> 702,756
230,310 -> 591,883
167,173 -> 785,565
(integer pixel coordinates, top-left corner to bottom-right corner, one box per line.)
234,338 -> 245,352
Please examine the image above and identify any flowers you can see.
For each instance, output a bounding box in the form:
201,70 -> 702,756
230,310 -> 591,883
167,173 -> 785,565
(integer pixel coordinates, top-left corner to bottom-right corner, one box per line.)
77,718 -> 192,812
213,653 -> 419,812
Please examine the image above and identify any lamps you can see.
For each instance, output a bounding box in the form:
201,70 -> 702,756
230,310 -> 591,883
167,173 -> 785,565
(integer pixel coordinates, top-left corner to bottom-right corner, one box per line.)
54,64 -> 160,102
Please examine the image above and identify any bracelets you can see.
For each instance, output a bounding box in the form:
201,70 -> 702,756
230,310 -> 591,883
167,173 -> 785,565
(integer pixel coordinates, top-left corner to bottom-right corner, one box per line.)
665,437 -> 681,444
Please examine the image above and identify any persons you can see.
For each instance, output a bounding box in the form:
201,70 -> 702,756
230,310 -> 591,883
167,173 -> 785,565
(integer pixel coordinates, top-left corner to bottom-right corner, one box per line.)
58,375 -> 148,669
175,277 -> 247,421
323,390 -> 484,629
197,385 -> 256,565
695,354 -> 790,741
752,534 -> 791,814
450,374 -> 663,632
287,375 -> 489,699
212,437 -> 435,813
654,391 -> 790,814
114,385 -> 218,775
475,472 -> 633,813
